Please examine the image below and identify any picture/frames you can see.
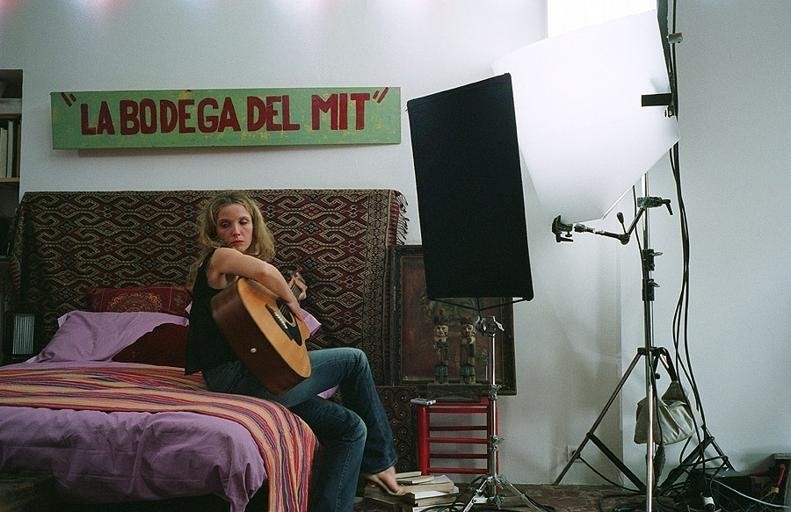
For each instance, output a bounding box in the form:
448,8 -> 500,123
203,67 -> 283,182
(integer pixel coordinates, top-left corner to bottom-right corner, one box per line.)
387,241 -> 519,398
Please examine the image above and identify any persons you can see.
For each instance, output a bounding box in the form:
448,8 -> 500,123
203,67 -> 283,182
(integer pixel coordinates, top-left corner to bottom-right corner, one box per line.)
459,313 -> 479,384
186,190 -> 409,510
433,307 -> 451,384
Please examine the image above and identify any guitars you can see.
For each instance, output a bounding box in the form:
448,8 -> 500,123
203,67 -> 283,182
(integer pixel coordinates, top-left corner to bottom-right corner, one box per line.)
211,272 -> 312,393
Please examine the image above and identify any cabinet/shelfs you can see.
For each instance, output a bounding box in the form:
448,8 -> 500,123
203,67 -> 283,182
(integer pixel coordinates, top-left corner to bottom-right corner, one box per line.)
0,112 -> 20,184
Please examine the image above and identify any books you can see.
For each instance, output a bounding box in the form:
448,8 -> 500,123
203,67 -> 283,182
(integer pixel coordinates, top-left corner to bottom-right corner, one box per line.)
362,464 -> 462,511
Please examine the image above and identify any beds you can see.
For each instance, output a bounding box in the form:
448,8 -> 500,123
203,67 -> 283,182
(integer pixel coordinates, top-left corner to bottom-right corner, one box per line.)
0,187 -> 410,512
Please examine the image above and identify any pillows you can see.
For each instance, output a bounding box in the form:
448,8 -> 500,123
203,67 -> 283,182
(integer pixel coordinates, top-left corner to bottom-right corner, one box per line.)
110,322 -> 188,369
81,281 -> 192,366
29,309 -> 189,364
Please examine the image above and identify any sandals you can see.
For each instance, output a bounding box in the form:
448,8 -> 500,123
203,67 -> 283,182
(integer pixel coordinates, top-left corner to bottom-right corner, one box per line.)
363,471 -> 405,497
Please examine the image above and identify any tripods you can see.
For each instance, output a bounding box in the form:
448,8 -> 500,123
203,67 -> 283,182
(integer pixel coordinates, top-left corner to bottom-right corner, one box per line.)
462,315 -> 543,512
554,172 -> 736,512
